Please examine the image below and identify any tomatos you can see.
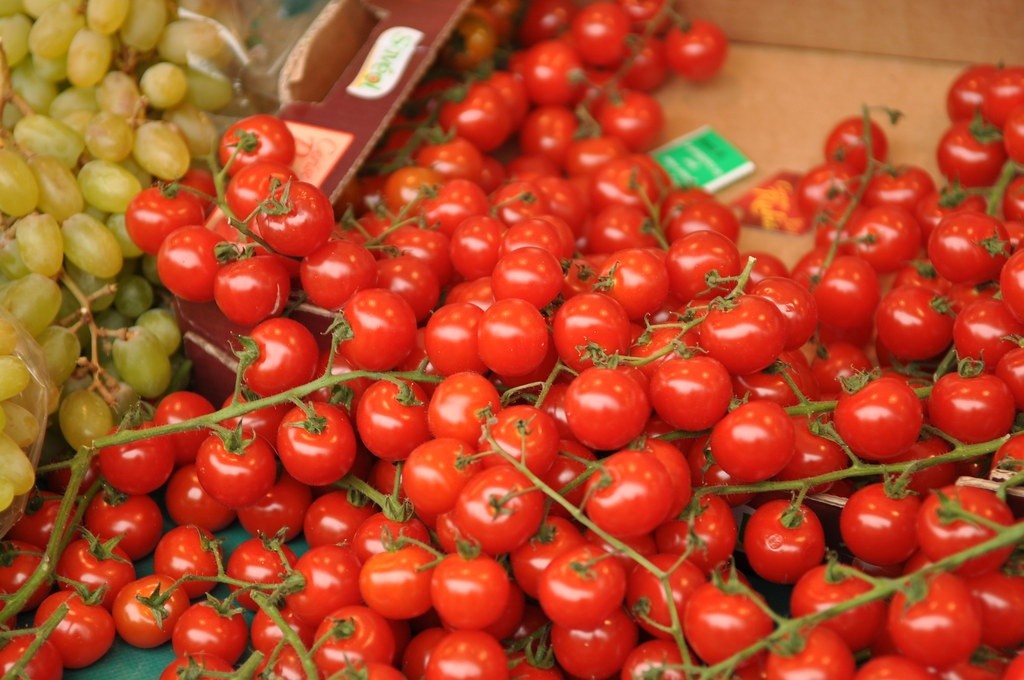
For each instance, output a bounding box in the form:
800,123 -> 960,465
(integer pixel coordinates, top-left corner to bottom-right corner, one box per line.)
0,0 -> 1024,679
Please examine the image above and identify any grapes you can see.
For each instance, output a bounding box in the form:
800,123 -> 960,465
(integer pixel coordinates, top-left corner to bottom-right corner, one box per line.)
1,0 -> 255,520
